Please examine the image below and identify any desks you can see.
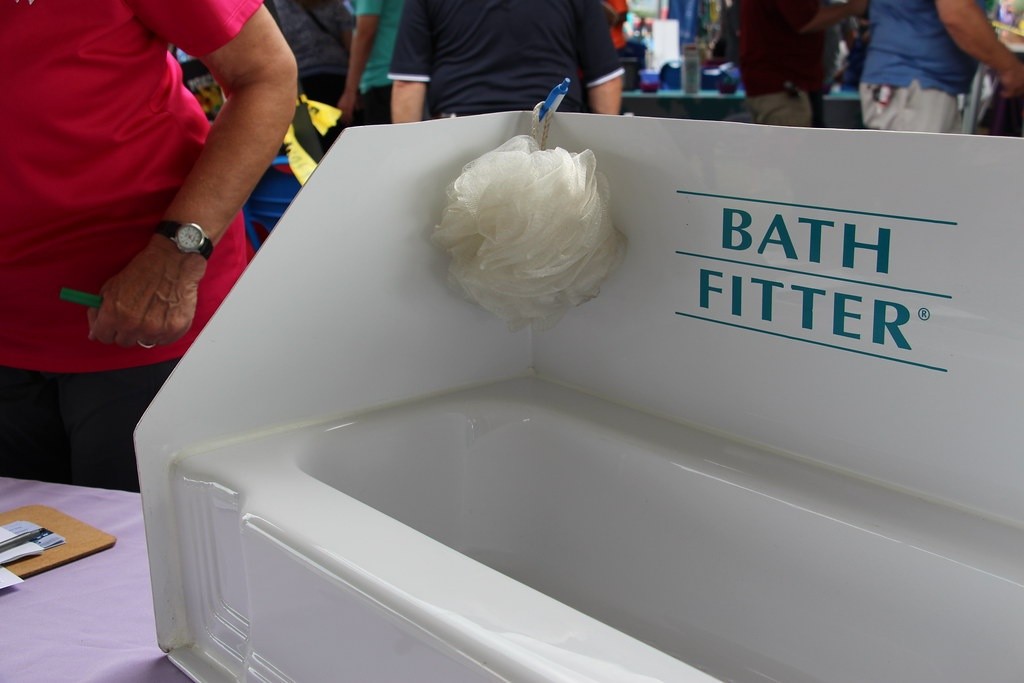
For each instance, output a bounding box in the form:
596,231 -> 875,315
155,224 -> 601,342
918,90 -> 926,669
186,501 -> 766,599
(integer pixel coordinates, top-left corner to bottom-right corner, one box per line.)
618,83 -> 871,131
0,476 -> 199,683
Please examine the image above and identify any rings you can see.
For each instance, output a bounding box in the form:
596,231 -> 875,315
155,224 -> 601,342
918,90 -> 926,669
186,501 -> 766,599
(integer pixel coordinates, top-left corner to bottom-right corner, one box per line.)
138,341 -> 156,349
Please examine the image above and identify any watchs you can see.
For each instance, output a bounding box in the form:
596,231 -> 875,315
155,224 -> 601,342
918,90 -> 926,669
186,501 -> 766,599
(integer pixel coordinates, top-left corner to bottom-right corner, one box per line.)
154,221 -> 213,260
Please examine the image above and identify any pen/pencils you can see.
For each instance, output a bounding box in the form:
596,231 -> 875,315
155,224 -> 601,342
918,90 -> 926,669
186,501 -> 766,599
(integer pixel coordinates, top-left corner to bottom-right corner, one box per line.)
59,288 -> 102,308
537,78 -> 571,124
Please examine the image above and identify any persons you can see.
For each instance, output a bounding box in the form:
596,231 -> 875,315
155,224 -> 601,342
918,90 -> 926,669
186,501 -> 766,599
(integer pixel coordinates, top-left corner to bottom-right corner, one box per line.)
1,0 -> 298,491
724,1 -> 741,61
819,0 -> 860,85
264,0 -> 355,159
858,0 -> 1024,133
738,0 -> 868,127
601,0 -> 629,58
164,0 -> 302,254
387,0 -> 625,125
338,0 -> 406,126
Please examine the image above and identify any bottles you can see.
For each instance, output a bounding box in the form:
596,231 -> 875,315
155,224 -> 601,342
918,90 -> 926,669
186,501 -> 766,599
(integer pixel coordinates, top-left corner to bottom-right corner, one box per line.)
681,43 -> 699,93
629,17 -> 657,70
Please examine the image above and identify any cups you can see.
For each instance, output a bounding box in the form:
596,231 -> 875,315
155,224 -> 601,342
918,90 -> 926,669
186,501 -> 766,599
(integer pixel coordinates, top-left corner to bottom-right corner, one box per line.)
639,70 -> 660,93
